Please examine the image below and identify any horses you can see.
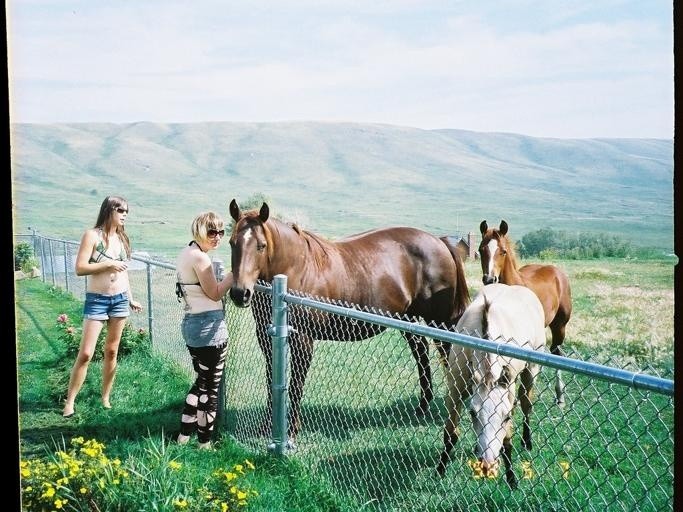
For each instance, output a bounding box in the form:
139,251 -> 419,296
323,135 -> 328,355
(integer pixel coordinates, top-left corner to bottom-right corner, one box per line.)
435,283 -> 546,491
478,219 -> 573,404
228,198 -> 472,444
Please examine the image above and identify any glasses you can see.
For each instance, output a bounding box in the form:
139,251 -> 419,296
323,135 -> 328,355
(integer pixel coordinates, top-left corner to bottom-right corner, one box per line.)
207,229 -> 224,238
112,206 -> 128,214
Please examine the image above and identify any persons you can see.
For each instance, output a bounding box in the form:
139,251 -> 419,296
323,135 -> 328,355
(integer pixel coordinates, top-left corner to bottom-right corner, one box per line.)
63,196 -> 141,418
175,212 -> 233,450
